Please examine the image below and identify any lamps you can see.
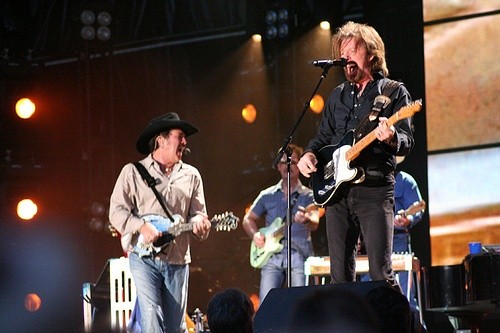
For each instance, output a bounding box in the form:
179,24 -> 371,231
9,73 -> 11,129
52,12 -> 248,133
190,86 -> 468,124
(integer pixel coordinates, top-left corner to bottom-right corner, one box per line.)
71,4 -> 120,52
260,0 -> 294,40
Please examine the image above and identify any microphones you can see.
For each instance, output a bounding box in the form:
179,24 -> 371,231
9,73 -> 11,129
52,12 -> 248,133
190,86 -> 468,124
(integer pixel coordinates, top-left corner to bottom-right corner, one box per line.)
185,143 -> 190,155
312,58 -> 347,67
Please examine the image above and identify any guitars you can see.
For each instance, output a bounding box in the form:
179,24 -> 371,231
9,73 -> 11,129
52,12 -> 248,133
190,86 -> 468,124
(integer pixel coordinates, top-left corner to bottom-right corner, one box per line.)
398,201 -> 425,218
250,205 -> 318,269
129,211 -> 239,259
311,100 -> 422,206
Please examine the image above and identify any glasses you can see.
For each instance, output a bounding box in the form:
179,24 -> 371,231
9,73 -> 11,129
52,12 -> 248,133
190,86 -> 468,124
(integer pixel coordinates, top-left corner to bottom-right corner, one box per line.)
278,159 -> 298,165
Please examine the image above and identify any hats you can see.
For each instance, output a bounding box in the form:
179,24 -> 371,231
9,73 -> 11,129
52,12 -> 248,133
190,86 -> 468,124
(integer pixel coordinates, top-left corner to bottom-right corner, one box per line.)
136,111 -> 199,155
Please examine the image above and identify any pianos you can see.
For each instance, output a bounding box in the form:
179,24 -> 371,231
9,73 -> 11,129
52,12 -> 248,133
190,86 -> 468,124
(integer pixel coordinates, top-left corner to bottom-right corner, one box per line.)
303,251 -> 423,324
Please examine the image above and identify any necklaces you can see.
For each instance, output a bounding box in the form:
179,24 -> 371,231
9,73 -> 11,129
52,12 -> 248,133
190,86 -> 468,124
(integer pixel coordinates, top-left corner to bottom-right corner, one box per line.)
166,168 -> 171,172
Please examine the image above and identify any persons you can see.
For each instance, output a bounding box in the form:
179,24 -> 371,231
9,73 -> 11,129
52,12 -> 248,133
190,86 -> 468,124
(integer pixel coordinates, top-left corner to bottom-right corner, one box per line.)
298,21 -> 414,295
242,144 -> 320,303
109,112 -> 211,333
206,289 -> 255,333
360,171 -> 425,323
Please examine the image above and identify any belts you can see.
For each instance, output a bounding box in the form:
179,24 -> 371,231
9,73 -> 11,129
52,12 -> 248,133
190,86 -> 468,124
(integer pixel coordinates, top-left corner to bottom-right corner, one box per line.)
349,166 -> 392,187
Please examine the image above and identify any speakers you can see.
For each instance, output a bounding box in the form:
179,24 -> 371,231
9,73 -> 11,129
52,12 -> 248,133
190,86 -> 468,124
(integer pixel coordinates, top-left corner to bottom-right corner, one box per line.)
252,280 -> 410,333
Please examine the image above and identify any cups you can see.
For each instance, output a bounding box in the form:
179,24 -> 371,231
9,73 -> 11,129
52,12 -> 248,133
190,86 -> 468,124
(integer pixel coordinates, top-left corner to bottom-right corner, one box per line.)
468,242 -> 482,254
